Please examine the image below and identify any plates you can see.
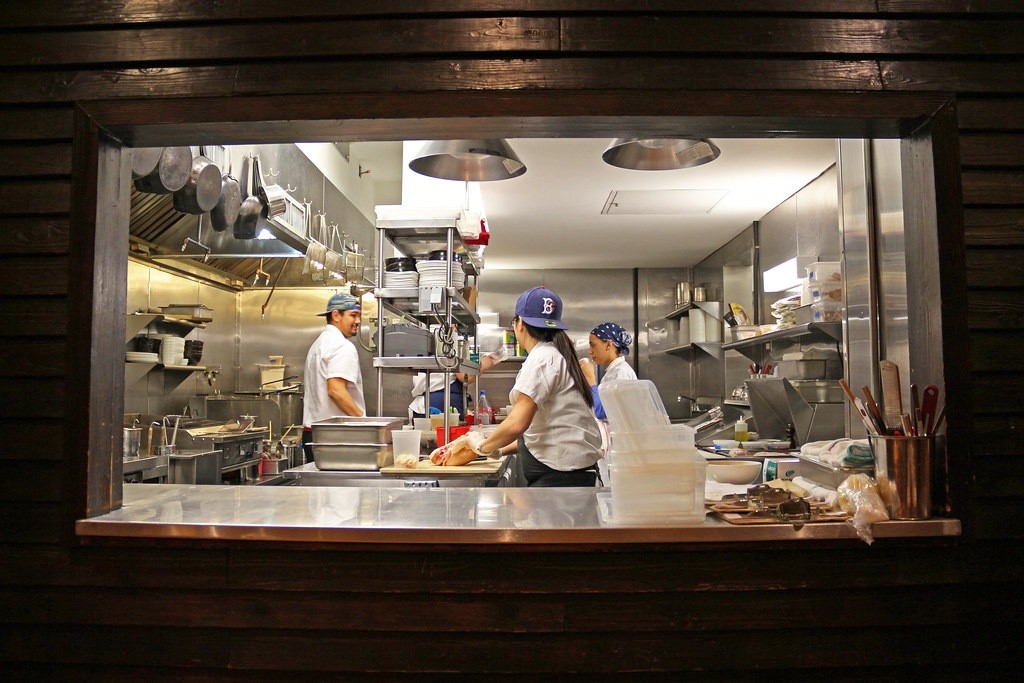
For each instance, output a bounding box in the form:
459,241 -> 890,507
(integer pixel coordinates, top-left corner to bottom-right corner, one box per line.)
126,352 -> 159,363
383,260 -> 465,289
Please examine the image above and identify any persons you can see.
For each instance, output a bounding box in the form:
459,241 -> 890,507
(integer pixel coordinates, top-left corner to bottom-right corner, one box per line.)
302,292 -> 367,463
578,322 -> 637,486
499,487 -> 589,526
442,285 -> 606,486
407,350 -> 504,428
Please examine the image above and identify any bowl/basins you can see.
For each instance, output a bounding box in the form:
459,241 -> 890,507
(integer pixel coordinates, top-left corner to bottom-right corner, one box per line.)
162,337 -> 204,366
383,250 -> 462,273
712,438 -> 792,450
731,386 -> 749,400
706,460 -> 763,484
805,262 -> 843,321
597,379 -> 707,524
269,355 -> 283,364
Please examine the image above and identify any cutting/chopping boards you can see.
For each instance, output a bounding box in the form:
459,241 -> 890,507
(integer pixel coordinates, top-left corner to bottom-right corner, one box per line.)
379,457 -> 505,473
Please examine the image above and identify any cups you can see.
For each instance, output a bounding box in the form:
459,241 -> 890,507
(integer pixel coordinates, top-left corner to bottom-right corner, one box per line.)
413,413 -> 460,430
665,282 -> 721,350
866,434 -> 946,520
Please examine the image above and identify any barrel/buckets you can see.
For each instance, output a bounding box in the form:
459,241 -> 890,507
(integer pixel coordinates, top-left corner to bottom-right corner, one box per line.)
391,430 -> 421,468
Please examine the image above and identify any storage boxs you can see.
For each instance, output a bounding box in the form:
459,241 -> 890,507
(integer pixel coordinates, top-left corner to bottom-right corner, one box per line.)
726,323 -> 757,342
304,439 -> 393,472
811,304 -> 843,322
254,363 -> 288,389
796,380 -> 844,405
436,425 -> 472,448
788,450 -> 874,491
303,415 -> 409,444
156,305 -> 214,320
804,260 -> 841,284
808,283 -> 842,304
772,358 -> 831,380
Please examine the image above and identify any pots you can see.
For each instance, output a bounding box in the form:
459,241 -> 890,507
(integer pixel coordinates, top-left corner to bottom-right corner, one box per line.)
132,145 -> 286,239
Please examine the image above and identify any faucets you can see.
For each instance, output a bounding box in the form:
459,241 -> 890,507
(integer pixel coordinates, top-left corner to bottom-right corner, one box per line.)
677,393 -> 696,404
260,375 -> 298,396
265,383 -> 303,396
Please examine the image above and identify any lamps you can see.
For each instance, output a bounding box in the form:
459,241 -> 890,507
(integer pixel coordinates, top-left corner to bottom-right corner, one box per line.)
408,138 -> 529,184
601,137 -> 723,172
761,254 -> 822,293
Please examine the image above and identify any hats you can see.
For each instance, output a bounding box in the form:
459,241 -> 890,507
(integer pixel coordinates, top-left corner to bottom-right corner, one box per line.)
515,285 -> 568,330
316,293 -> 361,316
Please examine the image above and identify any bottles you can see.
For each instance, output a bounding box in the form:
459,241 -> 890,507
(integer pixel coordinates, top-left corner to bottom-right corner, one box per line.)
478,390 -> 491,424
735,416 -> 748,441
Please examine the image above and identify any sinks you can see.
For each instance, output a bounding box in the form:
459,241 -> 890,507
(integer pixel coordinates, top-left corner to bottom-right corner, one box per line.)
671,418 -> 692,424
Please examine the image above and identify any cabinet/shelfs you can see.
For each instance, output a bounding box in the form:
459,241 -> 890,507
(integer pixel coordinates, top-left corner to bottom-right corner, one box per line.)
370,216 -> 481,449
130,310 -> 213,372
661,300 -> 720,362
717,322 -> 844,409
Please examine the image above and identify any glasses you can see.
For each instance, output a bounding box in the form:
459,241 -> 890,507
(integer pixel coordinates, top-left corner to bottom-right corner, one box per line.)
511,316 -> 518,328
